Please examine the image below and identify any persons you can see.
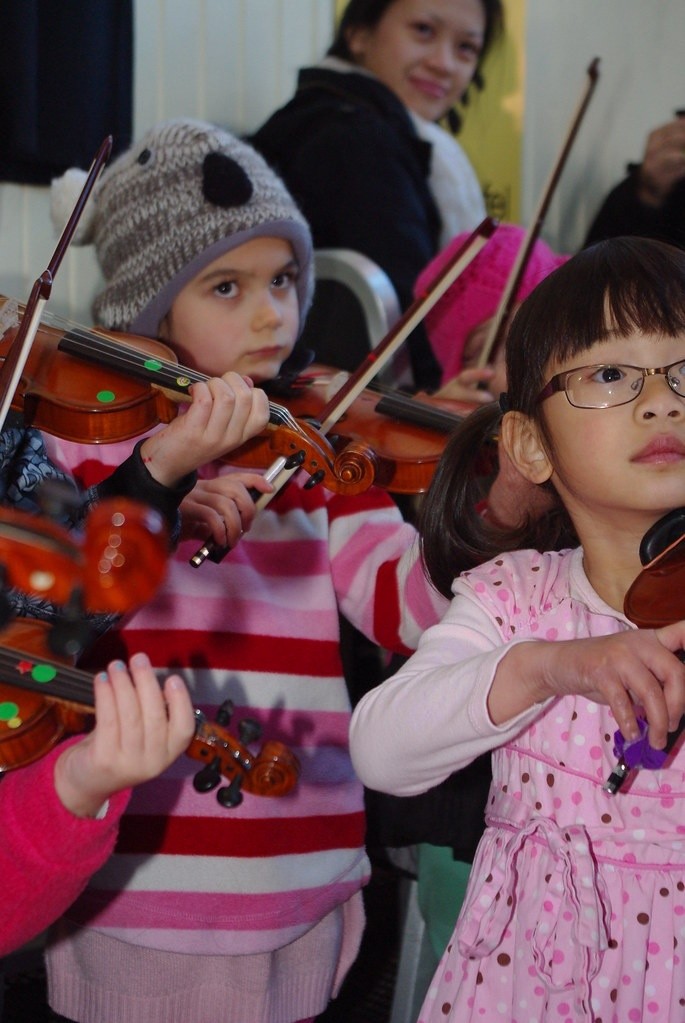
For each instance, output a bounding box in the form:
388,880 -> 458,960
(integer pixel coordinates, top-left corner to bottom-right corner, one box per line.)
25,119 -> 553,1023
575,106 -> 685,248
345,242 -> 684,1022
0,371 -> 270,651
241,0 -> 505,378
0,651 -> 195,960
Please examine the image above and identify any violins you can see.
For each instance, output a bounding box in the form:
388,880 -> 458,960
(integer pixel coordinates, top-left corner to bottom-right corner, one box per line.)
1,126 -> 522,618
602,507 -> 684,805
0,618 -> 303,808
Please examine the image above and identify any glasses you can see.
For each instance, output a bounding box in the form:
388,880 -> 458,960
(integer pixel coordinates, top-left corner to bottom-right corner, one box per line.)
533,359 -> 685,409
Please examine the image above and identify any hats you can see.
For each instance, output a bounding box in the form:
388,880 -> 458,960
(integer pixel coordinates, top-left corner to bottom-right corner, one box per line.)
50,116 -> 316,343
414,225 -> 572,390
